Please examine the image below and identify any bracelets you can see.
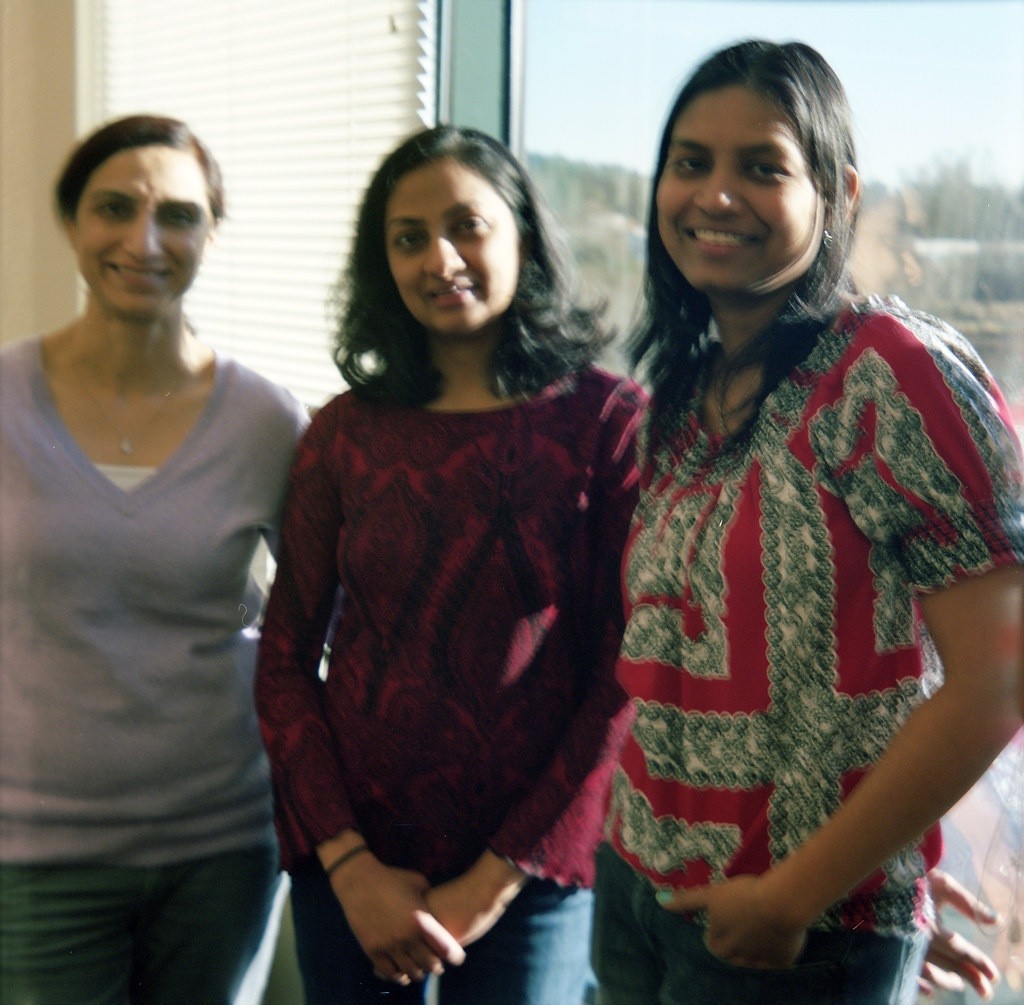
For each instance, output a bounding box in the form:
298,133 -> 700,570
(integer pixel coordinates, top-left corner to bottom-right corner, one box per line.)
326,842 -> 373,883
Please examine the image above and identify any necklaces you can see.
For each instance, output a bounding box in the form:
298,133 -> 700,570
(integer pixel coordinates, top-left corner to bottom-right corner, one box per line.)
68,317 -> 202,453
713,358 -> 763,444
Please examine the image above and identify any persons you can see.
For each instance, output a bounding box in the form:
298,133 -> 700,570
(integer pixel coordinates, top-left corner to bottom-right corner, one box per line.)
253,122 -> 655,1004
586,35 -> 1023,1005
0,117 -> 334,1005
